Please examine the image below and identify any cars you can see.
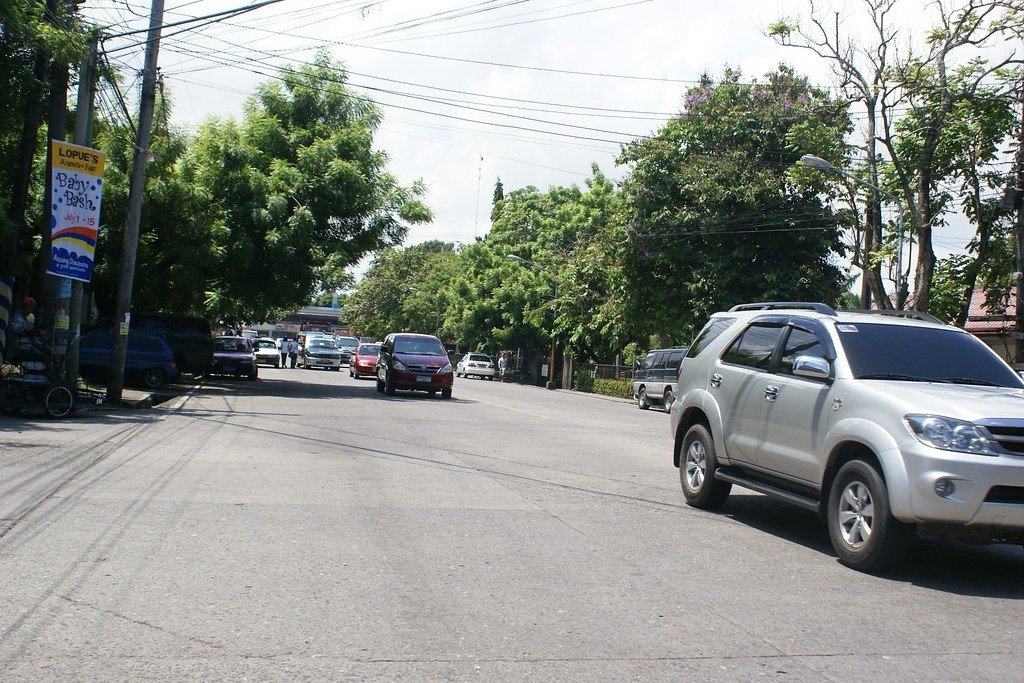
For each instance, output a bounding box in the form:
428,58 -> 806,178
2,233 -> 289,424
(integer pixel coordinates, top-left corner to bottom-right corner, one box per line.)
454,352 -> 496,382
76,325 -> 183,389
275,328 -> 385,379
201,335 -> 258,382
251,338 -> 280,368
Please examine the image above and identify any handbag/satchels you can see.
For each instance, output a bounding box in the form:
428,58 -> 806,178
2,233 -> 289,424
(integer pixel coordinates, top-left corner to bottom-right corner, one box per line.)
289,352 -> 294,358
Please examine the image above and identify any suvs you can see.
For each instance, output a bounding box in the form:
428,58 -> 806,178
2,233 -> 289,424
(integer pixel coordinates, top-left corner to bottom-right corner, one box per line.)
76,308 -> 213,378
634,345 -> 688,414
666,300 -> 1024,578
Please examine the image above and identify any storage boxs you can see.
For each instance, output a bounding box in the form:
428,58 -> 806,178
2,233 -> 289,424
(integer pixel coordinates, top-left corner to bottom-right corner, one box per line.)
13,331 -> 76,383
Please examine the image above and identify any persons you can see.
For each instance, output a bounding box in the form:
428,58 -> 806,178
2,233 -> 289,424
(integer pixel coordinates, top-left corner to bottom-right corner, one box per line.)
5,296 -> 53,363
290,337 -> 299,368
281,337 -> 289,368
498,353 -> 507,383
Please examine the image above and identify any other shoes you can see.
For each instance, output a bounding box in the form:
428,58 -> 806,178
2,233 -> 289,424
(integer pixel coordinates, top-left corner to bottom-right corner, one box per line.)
285,366 -> 287,368
282,366 -> 284,368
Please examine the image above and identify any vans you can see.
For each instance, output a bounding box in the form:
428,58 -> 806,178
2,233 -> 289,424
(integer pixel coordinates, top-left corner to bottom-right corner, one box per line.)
376,332 -> 455,399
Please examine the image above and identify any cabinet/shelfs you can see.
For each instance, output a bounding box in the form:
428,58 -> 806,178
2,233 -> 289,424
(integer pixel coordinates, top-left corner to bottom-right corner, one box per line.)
15,327 -> 69,381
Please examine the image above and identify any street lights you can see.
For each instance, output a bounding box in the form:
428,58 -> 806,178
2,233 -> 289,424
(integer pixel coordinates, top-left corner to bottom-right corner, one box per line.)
800,151 -> 904,316
507,253 -> 557,385
